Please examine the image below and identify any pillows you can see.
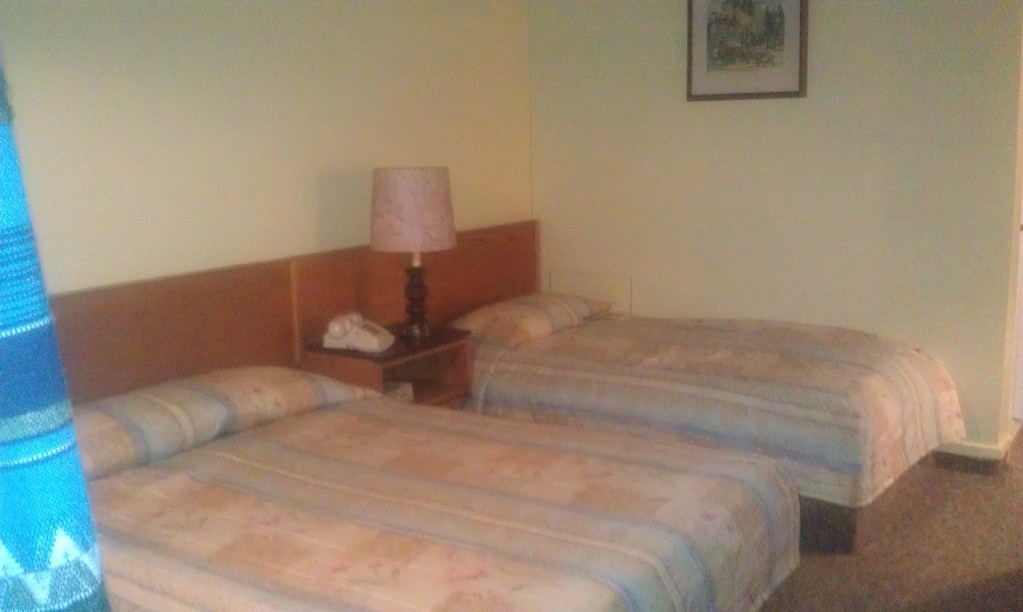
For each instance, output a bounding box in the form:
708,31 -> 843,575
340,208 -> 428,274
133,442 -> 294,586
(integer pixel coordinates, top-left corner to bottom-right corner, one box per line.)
449,292 -> 612,351
73,363 -> 383,481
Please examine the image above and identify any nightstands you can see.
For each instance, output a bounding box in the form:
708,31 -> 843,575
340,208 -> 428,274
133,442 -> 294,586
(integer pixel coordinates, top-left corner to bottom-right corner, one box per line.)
301,321 -> 475,411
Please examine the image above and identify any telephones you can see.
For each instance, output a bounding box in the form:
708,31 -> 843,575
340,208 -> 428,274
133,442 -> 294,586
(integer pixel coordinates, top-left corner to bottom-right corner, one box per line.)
322,314 -> 395,352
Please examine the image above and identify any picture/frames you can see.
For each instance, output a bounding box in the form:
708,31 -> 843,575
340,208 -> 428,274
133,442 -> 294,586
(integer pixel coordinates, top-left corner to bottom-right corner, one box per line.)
687,0 -> 810,102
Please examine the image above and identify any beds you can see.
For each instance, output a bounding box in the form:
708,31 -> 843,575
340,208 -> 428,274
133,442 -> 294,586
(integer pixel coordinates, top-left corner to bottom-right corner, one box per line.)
49,257 -> 801,612
401,218 -> 969,556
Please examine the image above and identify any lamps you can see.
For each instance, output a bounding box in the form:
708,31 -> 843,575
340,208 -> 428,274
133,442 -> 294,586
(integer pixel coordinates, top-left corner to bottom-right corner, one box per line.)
370,165 -> 457,349
